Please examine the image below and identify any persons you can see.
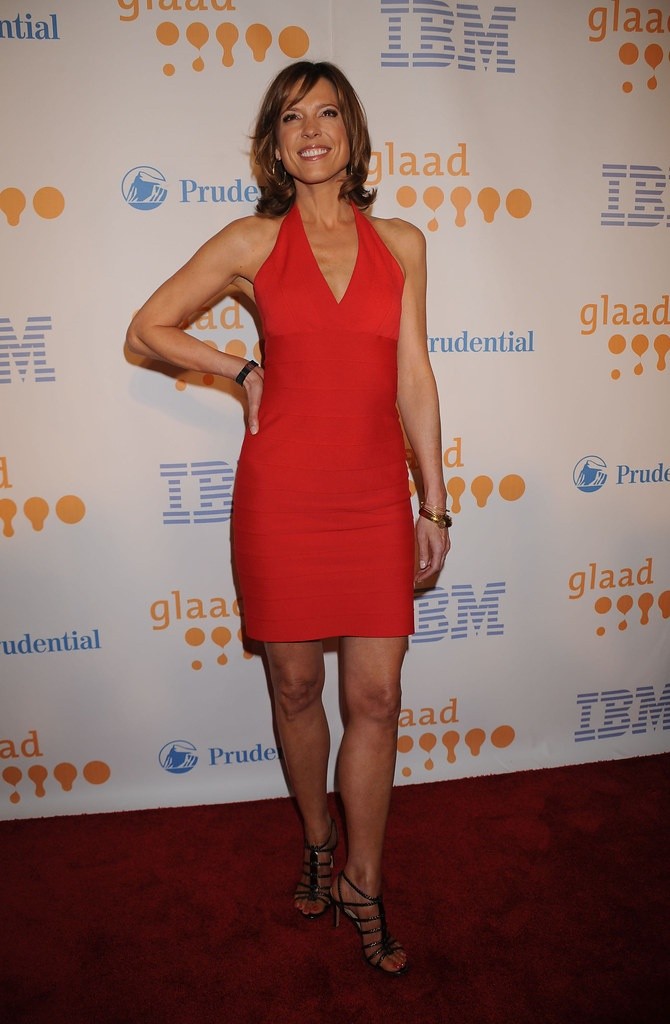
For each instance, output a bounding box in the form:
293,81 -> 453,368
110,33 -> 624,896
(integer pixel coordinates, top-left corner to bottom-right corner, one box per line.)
126,63 -> 451,975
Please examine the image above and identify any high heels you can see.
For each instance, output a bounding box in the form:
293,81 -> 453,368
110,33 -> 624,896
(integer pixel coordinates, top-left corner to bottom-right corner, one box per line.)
293,817 -> 338,919
328,870 -> 410,977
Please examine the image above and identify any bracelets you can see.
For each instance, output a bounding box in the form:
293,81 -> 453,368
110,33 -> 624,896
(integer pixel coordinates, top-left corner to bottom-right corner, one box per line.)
418,502 -> 452,529
236,360 -> 259,387
